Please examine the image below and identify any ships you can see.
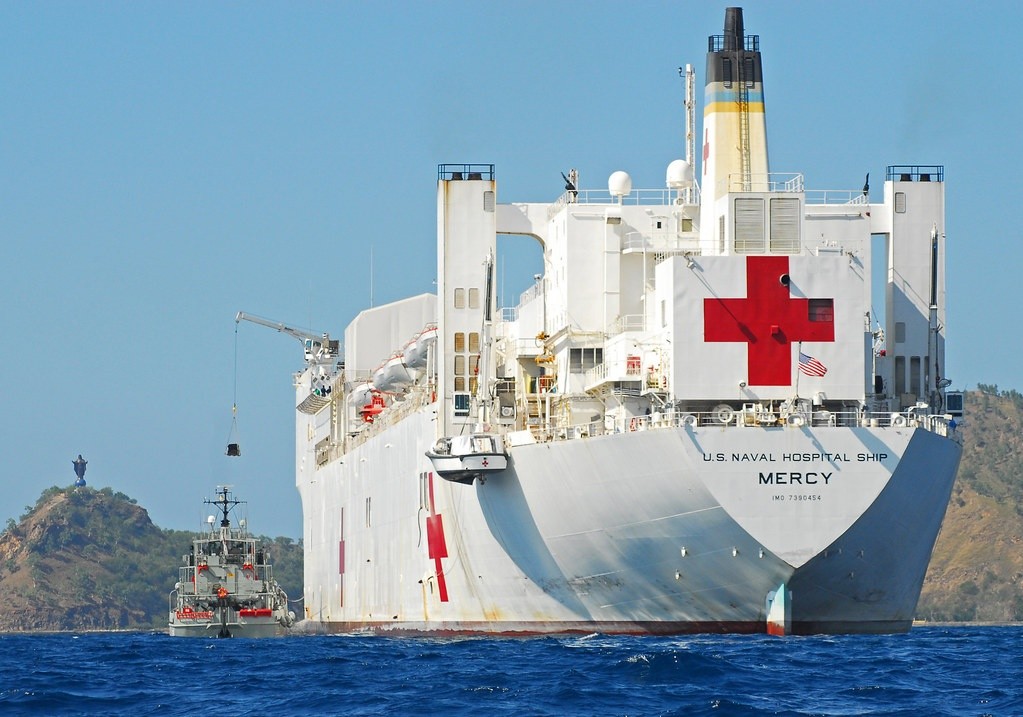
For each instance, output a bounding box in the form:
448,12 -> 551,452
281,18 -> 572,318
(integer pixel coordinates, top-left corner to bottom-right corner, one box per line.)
292,7 -> 965,636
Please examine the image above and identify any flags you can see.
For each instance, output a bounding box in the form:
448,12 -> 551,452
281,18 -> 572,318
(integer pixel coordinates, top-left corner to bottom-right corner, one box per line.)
798,352 -> 827,377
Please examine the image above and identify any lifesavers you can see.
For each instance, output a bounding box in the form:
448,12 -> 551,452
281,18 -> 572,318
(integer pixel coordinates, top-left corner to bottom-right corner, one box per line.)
218,588 -> 227,598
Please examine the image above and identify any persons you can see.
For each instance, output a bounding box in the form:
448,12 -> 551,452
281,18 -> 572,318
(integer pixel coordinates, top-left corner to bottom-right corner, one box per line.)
327,386 -> 331,394
77,455 -> 86,479
315,388 -> 320,396
321,385 -> 327,397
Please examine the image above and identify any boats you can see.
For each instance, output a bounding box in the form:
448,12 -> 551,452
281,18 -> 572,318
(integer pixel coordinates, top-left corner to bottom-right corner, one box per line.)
402,338 -> 427,368
167,486 -> 296,638
425,432 -> 509,485
359,395 -> 386,422
385,355 -> 417,383
372,366 -> 407,394
415,327 -> 438,358
353,384 -> 374,406
